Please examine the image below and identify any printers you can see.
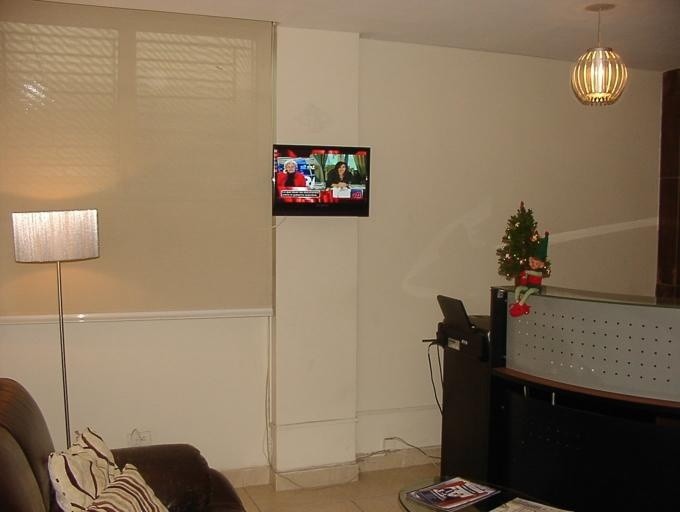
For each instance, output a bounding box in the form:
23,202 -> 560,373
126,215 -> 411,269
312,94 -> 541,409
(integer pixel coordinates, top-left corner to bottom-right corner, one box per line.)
421,294 -> 491,362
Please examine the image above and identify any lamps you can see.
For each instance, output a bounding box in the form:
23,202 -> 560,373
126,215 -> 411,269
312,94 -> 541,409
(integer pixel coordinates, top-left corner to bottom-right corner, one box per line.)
11,207 -> 100,449
571,2 -> 629,106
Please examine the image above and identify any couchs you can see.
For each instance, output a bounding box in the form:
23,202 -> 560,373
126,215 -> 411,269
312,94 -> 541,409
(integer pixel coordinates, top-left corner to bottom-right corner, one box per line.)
1,376 -> 248,510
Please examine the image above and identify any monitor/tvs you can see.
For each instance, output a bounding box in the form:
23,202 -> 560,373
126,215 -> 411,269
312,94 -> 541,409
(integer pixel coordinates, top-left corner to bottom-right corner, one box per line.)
271,144 -> 371,218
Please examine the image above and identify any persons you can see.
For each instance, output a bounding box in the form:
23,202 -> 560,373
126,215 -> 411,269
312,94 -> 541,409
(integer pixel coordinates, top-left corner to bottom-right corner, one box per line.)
277,159 -> 306,187
326,162 -> 352,187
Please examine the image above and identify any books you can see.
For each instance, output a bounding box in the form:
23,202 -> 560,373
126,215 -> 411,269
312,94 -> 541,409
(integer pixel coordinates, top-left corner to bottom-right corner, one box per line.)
405,477 -> 500,512
